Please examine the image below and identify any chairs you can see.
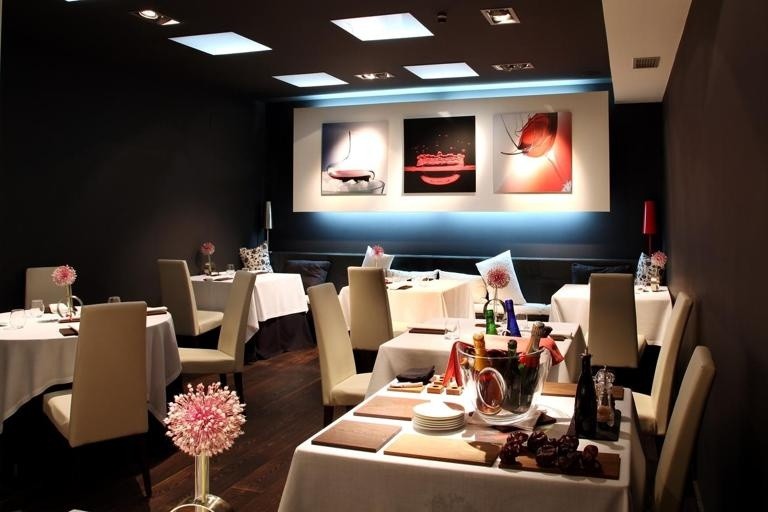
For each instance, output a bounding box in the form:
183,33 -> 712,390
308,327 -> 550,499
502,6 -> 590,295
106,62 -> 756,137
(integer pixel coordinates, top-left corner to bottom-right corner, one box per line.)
174,271 -> 256,403
632,292 -> 694,436
23,267 -> 69,309
654,346 -> 717,509
590,272 -> 647,370
42,300 -> 152,497
158,260 -> 223,346
349,266 -> 393,351
305,283 -> 373,429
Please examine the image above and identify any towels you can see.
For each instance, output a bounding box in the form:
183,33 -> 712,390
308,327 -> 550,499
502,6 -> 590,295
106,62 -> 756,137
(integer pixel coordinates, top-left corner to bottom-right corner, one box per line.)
397,366 -> 436,385
398,286 -> 413,289
59,328 -> 77,336
467,407 -> 542,431
215,277 -> 234,281
409,328 -> 444,335
469,410 -> 556,434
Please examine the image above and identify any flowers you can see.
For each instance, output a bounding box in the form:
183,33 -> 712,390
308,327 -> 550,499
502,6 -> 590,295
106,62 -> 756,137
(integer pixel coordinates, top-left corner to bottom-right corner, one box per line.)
52,265 -> 76,286
164,382 -> 246,458
651,251 -> 668,267
201,242 -> 215,255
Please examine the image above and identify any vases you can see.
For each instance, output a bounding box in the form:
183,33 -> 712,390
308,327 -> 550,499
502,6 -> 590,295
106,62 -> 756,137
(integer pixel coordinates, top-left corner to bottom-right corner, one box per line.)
207,255 -> 212,271
655,266 -> 659,279
376,257 -> 378,267
66,284 -> 73,310
372,245 -> 384,258
192,454 -> 211,512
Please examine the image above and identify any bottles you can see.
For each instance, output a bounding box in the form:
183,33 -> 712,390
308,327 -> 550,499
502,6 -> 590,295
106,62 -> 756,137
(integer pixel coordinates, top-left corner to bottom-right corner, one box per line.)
505,299 -> 521,337
519,322 -> 545,410
458,358 -> 476,400
573,353 -> 598,438
471,334 -> 498,413
485,310 -> 498,335
504,340 -> 519,404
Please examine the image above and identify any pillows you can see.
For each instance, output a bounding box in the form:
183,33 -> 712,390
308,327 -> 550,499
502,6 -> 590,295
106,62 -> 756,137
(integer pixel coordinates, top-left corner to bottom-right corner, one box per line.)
362,245 -> 394,268
437,273 -> 490,304
475,250 -> 527,304
572,264 -> 631,284
634,252 -> 660,286
285,260 -> 330,292
239,242 -> 273,272
389,270 -> 439,281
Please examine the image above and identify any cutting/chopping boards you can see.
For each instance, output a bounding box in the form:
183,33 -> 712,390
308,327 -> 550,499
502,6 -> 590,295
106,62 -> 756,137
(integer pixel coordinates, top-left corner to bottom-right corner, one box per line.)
384,427 -> 502,467
360,391 -> 415,420
312,419 -> 400,452
539,378 -> 627,398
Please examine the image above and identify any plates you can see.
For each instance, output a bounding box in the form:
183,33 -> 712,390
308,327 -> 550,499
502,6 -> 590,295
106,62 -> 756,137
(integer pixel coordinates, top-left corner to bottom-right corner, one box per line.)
412,401 -> 466,431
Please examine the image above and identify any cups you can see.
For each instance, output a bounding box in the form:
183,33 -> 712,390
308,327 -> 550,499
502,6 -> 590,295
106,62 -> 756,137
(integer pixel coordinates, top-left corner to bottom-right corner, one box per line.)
28,300 -> 46,325
225,263 -> 233,273
445,319 -> 460,341
203,262 -> 214,273
9,308 -> 26,332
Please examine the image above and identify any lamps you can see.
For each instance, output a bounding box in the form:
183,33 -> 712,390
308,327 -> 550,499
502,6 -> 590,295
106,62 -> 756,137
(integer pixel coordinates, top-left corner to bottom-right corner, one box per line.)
642,201 -> 656,252
266,201 -> 272,242
